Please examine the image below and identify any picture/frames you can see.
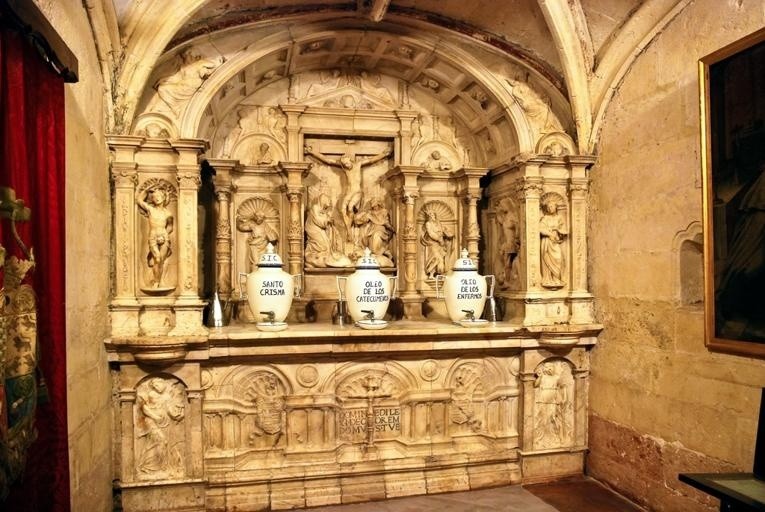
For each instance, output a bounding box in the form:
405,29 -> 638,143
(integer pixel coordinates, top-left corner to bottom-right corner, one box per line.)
698,26 -> 765,359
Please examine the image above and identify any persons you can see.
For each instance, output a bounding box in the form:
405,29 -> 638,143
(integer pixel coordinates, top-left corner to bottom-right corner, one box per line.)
141,375 -> 184,470
496,198 -> 520,290
153,46 -> 215,117
304,146 -> 394,267
540,202 -> 569,287
137,184 -> 174,288
236,211 -> 278,273
534,363 -> 568,447
504,68 -> 569,135
420,210 -> 455,280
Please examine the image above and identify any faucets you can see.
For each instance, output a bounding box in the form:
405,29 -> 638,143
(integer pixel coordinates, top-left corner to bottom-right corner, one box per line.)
259,311 -> 276,325
360,309 -> 375,323
461,309 -> 475,322
